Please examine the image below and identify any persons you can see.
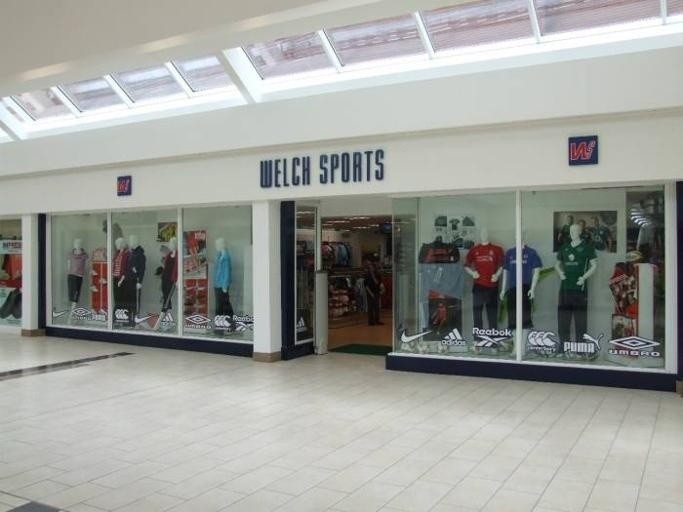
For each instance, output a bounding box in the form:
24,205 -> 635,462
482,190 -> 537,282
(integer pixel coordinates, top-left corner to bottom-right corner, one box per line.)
362,261 -> 385,326
110,237 -> 130,323
416,223 -> 599,358
155,236 -> 177,335
213,237 -> 236,336
65,229 -> 88,328
122,235 -> 145,329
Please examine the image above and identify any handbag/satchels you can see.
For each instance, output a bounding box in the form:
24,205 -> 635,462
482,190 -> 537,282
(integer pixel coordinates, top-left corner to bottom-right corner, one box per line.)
417,235 -> 461,264
